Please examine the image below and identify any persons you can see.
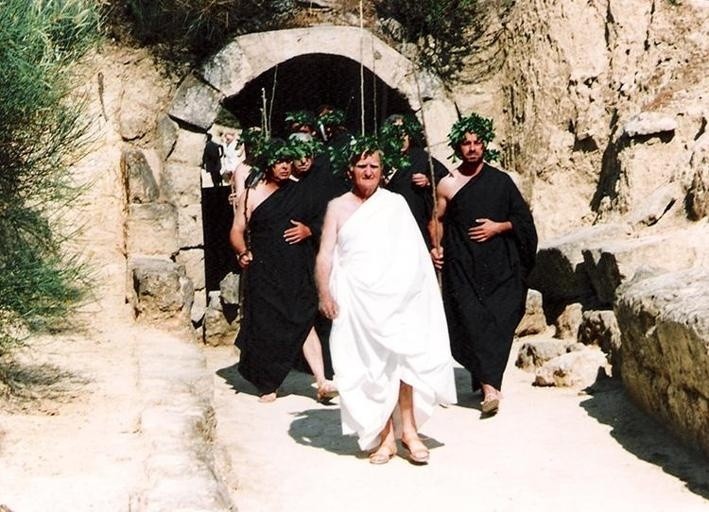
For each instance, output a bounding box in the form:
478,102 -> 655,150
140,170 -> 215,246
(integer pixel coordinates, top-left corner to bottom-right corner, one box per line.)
228,137 -> 339,403
313,132 -> 431,467
428,110 -> 540,416
203,107 -> 452,251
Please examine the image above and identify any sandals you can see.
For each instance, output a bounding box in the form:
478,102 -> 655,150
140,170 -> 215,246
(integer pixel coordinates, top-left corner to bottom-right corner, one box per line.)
400,432 -> 430,462
317,383 -> 340,404
259,390 -> 277,403
481,391 -> 504,416
368,441 -> 398,464
